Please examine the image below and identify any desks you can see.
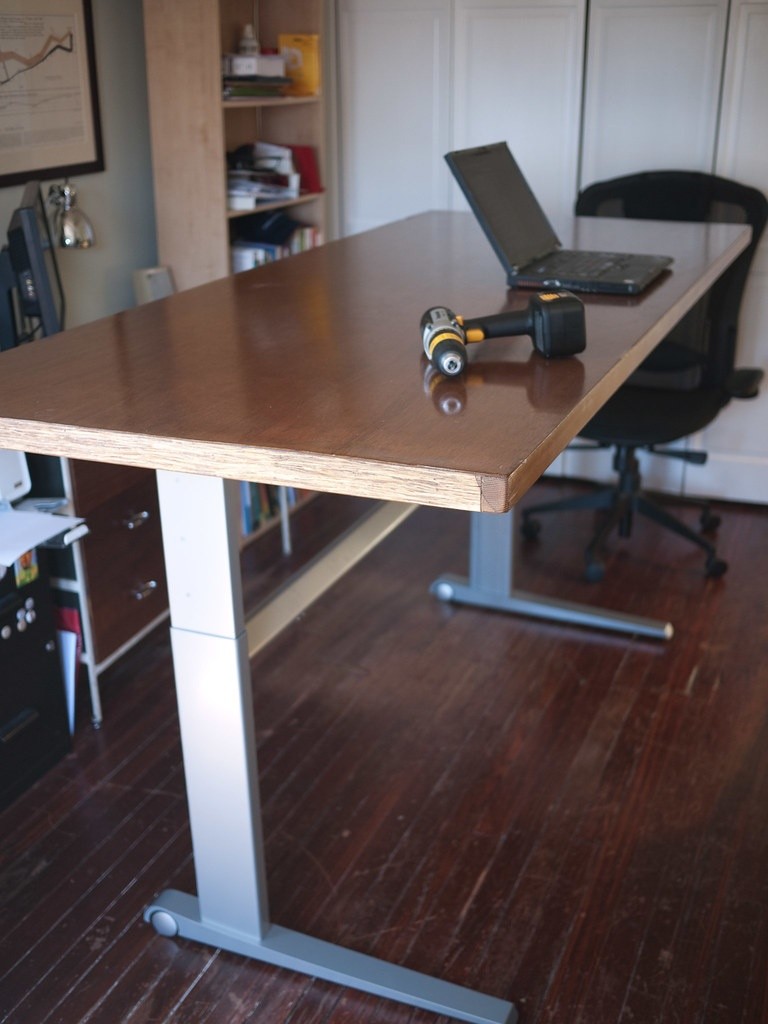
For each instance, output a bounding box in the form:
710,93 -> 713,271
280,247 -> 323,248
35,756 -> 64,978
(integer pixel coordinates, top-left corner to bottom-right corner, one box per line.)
0,207 -> 754,1024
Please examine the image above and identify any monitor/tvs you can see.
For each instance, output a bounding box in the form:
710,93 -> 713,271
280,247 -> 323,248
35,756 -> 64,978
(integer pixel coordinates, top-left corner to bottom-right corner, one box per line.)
0,179 -> 66,352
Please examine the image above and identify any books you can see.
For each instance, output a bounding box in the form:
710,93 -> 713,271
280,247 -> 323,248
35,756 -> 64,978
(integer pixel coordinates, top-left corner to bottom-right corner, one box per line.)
240,482 -> 309,536
231,139 -> 324,210
235,222 -> 322,274
222,76 -> 293,100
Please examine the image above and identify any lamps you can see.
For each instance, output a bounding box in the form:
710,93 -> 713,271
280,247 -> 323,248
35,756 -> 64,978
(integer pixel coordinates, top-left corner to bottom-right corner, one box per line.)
49,183 -> 97,249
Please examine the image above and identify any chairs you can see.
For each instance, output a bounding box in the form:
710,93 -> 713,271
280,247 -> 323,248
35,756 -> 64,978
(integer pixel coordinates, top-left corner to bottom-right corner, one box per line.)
522,171 -> 768,585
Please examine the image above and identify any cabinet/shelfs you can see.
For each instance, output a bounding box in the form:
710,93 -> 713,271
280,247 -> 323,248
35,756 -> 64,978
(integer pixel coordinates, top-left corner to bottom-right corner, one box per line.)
143,0 -> 329,548
35,454 -> 171,730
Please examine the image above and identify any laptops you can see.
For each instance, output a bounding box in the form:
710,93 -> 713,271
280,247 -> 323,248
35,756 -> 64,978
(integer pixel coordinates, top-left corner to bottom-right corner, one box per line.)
444,140 -> 673,294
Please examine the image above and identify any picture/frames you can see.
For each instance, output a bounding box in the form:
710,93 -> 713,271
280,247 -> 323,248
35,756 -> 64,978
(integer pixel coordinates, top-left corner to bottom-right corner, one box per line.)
0,0 -> 106,188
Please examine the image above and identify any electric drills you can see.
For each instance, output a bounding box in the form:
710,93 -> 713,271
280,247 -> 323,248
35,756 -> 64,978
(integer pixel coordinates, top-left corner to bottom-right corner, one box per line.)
421,287 -> 587,376
422,352 -> 585,417
